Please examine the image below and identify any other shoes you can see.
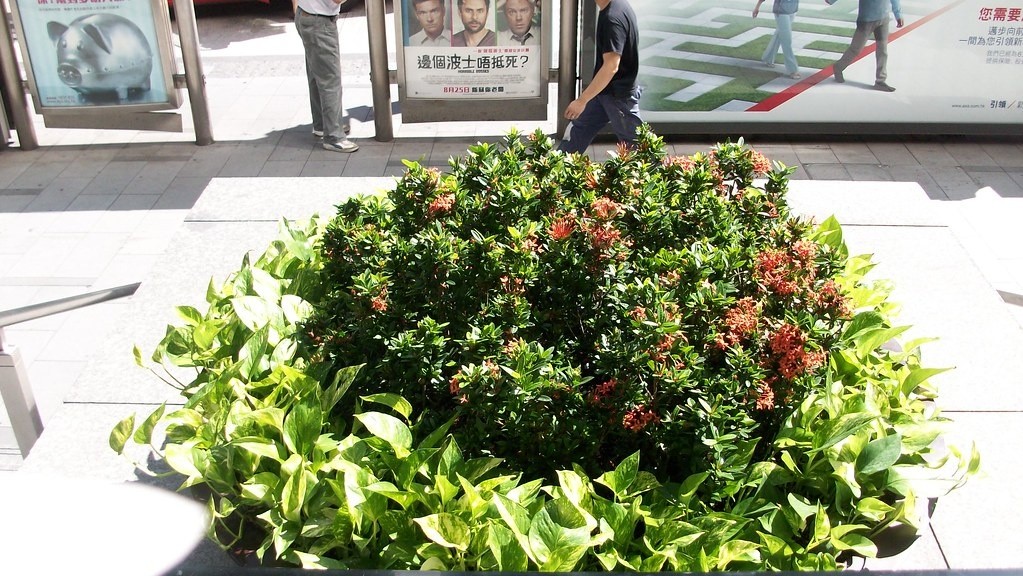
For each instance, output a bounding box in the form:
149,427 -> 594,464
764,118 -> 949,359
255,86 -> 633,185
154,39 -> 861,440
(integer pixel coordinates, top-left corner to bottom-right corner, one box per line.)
833,62 -> 844,82
873,82 -> 895,92
789,72 -> 800,79
766,61 -> 777,67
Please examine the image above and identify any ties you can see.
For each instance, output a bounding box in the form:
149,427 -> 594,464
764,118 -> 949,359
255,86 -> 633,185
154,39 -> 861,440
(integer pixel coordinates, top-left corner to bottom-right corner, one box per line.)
512,32 -> 532,45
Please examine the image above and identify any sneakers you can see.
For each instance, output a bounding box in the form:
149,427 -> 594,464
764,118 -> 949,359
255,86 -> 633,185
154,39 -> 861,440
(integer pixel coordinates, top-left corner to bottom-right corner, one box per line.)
313,124 -> 349,136
322,139 -> 358,152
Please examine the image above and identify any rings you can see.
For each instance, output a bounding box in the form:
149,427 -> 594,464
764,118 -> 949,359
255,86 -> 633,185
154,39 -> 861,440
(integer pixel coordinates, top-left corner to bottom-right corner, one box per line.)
572,115 -> 575,118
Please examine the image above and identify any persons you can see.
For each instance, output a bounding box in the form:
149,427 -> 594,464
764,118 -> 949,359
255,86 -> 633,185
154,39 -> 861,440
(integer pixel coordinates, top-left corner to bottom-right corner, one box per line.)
825,0 -> 904,91
293,1 -> 360,153
496,0 -> 540,47
409,0 -> 452,47
556,0 -> 646,155
752,0 -> 801,79
451,0 -> 495,46
